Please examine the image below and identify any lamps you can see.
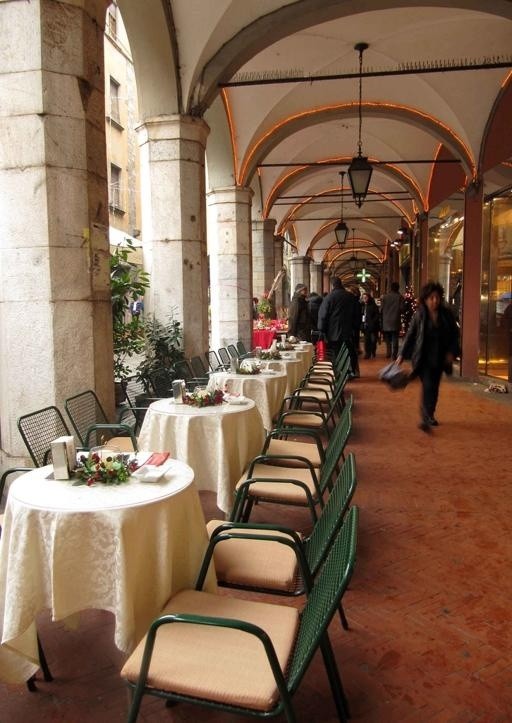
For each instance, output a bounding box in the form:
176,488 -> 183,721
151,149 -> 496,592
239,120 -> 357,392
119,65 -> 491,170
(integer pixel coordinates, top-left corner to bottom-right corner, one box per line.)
390,225 -> 409,248
330,168 -> 349,250
349,40 -> 374,207
349,228 -> 361,283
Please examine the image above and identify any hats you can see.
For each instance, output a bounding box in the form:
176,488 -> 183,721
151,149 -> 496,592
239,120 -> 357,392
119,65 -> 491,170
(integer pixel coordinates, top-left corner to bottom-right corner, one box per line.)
295,284 -> 307,293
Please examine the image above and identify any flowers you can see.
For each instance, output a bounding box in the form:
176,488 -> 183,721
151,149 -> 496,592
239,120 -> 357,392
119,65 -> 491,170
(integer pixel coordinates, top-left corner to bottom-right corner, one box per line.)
253,287 -> 272,312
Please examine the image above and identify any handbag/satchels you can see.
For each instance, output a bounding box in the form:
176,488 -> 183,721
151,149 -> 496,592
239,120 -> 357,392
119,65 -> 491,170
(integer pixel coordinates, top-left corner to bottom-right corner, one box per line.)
378,363 -> 410,391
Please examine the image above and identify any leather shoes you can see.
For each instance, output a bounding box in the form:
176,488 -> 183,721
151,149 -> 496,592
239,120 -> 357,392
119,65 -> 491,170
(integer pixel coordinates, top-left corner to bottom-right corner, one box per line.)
429,415 -> 438,426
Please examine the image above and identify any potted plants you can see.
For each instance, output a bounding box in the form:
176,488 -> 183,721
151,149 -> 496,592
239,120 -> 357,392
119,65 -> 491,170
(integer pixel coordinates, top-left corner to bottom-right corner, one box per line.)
109,234 -> 151,404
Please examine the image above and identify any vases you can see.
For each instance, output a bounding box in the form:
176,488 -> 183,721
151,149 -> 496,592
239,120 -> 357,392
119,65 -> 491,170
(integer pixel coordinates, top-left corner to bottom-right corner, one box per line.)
258,310 -> 265,320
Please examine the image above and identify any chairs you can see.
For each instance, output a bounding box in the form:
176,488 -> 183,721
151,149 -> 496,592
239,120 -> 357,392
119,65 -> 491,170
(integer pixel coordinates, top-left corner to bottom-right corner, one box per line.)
0,334 -> 366,722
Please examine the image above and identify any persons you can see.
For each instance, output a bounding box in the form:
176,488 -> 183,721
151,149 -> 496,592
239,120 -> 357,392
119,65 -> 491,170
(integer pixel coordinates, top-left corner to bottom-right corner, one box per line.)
502,299 -> 511,379
130,296 -> 141,321
253,297 -> 258,321
287,277 -> 462,431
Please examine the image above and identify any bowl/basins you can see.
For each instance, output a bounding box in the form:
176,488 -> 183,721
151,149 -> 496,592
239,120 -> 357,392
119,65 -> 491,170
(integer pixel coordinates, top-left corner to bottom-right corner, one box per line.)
194,385 -> 210,393
88,444 -> 121,458
130,463 -> 172,483
